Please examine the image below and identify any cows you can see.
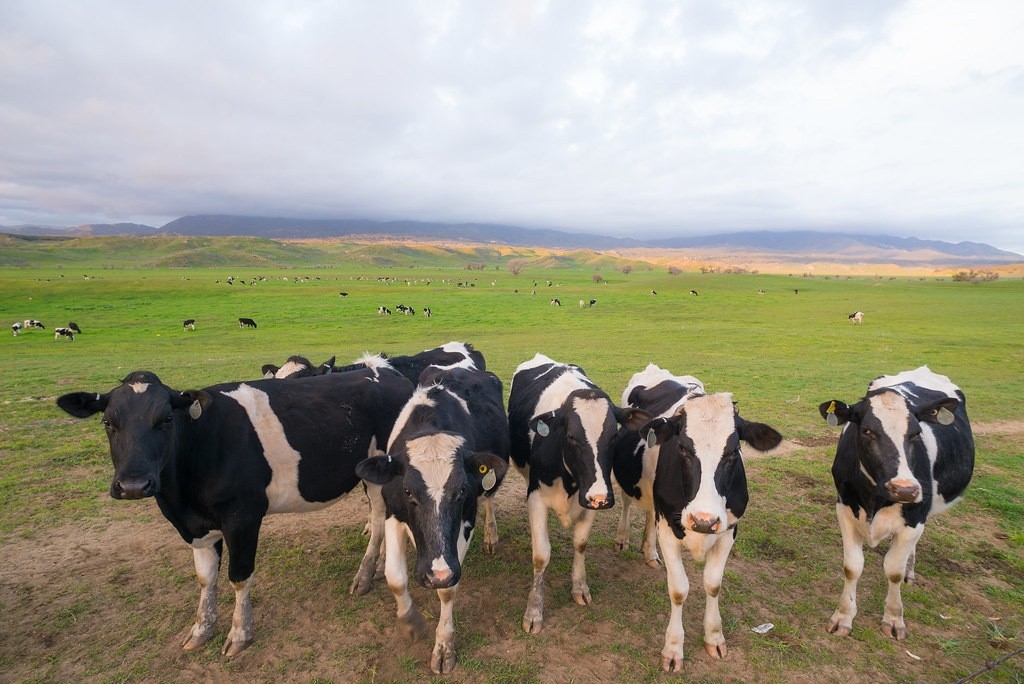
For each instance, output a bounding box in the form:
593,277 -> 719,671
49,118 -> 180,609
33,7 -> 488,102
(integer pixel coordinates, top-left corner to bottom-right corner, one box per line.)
356,342 -> 509,675
9,270 -> 864,335
816,363 -> 977,642
612,362 -> 784,665
55,342 -> 486,657
507,352 -> 654,635
